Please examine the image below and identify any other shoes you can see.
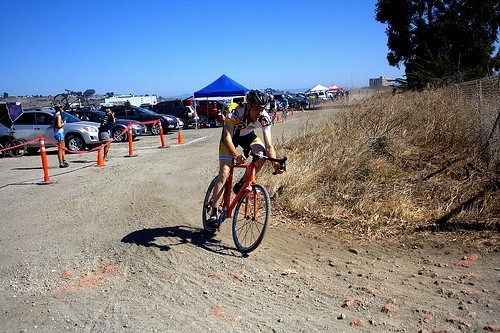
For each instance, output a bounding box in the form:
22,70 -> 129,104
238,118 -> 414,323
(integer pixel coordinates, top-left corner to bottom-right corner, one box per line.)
59,164 -> 67,168
63,163 -> 69,166
104,156 -> 111,161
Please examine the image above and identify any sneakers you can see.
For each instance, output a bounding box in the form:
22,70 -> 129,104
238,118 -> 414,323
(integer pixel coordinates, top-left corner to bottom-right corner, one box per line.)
206,217 -> 219,228
233,183 -> 245,195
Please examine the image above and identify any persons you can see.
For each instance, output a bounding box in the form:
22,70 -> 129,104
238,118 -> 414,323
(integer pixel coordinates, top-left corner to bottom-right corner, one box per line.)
268,95 -> 277,120
207,89 -> 285,229
52,105 -> 69,167
98,108 -> 115,162
279,95 -> 289,116
328,88 -> 349,103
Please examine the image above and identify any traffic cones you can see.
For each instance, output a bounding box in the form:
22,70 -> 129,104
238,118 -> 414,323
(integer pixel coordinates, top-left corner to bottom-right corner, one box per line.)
176,131 -> 186,144
276,113 -> 282,123
321,103 -> 323,107
301,106 -> 304,113
94,148 -> 106,167
313,105 -> 315,110
291,109 -> 295,116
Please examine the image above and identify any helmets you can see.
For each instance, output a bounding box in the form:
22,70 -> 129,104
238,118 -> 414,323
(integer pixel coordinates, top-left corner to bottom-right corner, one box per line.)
55,105 -> 62,108
246,90 -> 268,108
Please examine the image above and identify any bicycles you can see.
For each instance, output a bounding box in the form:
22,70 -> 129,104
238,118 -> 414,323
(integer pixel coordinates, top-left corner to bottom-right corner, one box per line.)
201,142 -> 288,254
53,88 -> 91,111
0,130 -> 27,157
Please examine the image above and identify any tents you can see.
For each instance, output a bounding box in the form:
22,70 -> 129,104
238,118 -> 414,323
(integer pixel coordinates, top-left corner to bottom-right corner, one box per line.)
193,74 -> 251,130
310,85 -> 328,93
328,84 -> 341,91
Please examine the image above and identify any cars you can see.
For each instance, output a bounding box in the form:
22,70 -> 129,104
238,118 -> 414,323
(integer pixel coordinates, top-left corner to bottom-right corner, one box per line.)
0,108 -> 110,153
66,92 -> 317,143
